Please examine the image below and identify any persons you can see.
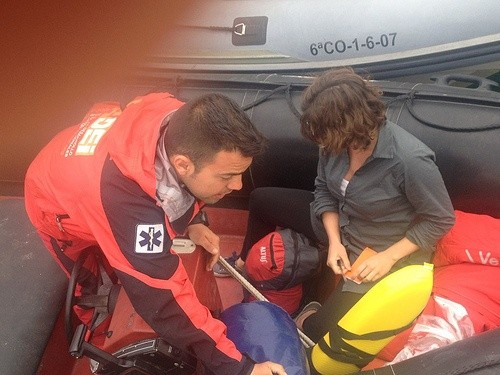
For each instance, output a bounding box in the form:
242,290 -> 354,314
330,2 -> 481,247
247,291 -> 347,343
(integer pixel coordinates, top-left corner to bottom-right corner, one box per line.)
24,92 -> 287,375
212,69 -> 455,344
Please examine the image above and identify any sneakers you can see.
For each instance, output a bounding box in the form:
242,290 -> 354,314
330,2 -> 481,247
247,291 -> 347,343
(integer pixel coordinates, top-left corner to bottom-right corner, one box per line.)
294,301 -> 321,335
213,251 -> 242,277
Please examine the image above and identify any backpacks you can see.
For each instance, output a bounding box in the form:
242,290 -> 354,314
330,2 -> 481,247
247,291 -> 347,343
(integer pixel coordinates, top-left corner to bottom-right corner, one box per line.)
243,226 -> 323,314
